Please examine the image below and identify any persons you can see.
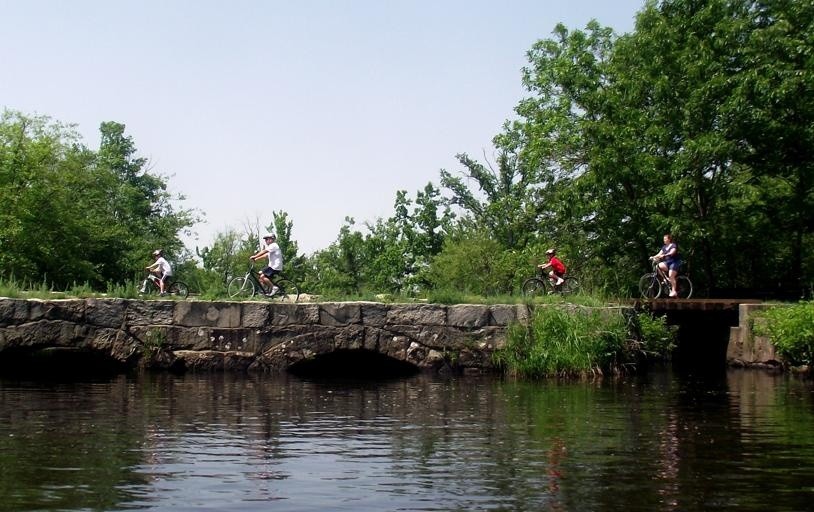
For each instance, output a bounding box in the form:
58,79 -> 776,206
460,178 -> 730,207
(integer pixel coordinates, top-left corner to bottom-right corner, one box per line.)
145,250 -> 172,297
648,234 -> 679,297
537,249 -> 566,296
249,232 -> 283,297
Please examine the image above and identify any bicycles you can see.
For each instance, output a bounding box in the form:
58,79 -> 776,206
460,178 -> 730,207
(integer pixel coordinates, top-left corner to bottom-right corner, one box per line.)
522,266 -> 578,297
136,269 -> 189,298
639,258 -> 693,299
227,258 -> 300,303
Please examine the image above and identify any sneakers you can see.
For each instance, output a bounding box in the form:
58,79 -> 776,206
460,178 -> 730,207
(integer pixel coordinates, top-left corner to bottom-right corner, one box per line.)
555,278 -> 564,285
269,286 -> 279,297
669,291 -> 677,297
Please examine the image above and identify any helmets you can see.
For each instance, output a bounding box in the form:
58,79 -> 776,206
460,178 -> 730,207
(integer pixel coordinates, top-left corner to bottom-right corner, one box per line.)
545,248 -> 555,254
263,233 -> 276,239
152,249 -> 162,255
